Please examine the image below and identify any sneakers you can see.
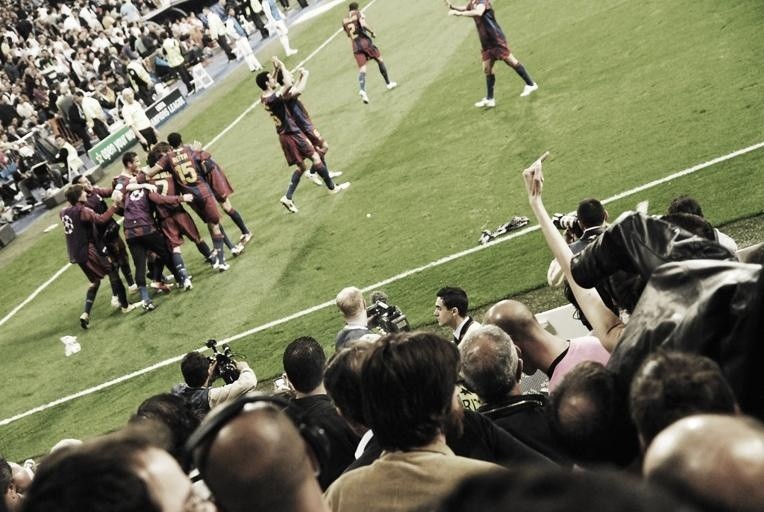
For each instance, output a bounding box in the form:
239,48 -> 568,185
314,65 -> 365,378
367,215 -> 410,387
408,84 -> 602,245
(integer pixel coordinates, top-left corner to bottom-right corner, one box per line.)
79,312 -> 90,329
474,96 -> 496,108
218,263 -> 230,271
155,283 -> 174,294
231,242 -> 245,256
141,300 -> 156,313
128,283 -> 148,295
328,181 -> 351,195
150,281 -> 171,293
304,170 -> 323,187
520,82 -> 539,97
182,276 -> 193,292
210,256 -> 226,269
386,82 -> 397,89
240,231 -> 253,244
111,295 -> 121,307
279,195 -> 299,214
120,302 -> 135,314
328,171 -> 343,179
359,90 -> 369,104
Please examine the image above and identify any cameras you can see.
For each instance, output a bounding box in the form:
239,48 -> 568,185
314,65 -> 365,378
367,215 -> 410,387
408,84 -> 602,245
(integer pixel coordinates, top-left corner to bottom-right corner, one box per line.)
364,300 -> 412,336
552,210 -> 583,239
205,338 -> 246,385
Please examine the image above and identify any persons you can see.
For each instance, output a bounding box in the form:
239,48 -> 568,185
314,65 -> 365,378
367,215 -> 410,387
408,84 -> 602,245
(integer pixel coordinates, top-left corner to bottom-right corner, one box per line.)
454,323 -> 573,466
427,466 -> 697,512
517,150 -> 744,354
53,134 -> 98,184
0,459 -> 36,511
264,54 -> 344,188
443,0 -> 538,108
200,6 -> 237,63
550,362 -> 645,480
254,56 -> 350,213
159,31 -> 196,97
660,195 -> 739,253
69,174 -> 139,308
59,184 -> 142,328
322,330 -> 503,512
279,337 -> 359,490
146,152 -> 218,274
118,1 -> 157,53
194,394 -> 334,512
120,182 -> 193,308
332,284 -> 385,350
225,5 -> 263,73
322,344 -> 559,468
139,134 -> 246,275
483,299 -> 609,391
127,394 -> 202,455
629,346 -> 743,448
175,351 -> 257,420
111,150 -> 180,285
433,286 -> 482,345
640,411 -> 762,512
0,122 -> 29,223
26,121 -> 61,171
0,0 -> 75,121
261,1 -> 298,56
75,0 -> 116,92
117,53 -> 155,106
319,340 -> 382,473
340,2 -> 396,103
74,92 -> 111,140
159,10 -> 208,44
120,88 -> 159,151
177,141 -> 252,246
545,197 -> 623,313
19,423 -> 218,512
